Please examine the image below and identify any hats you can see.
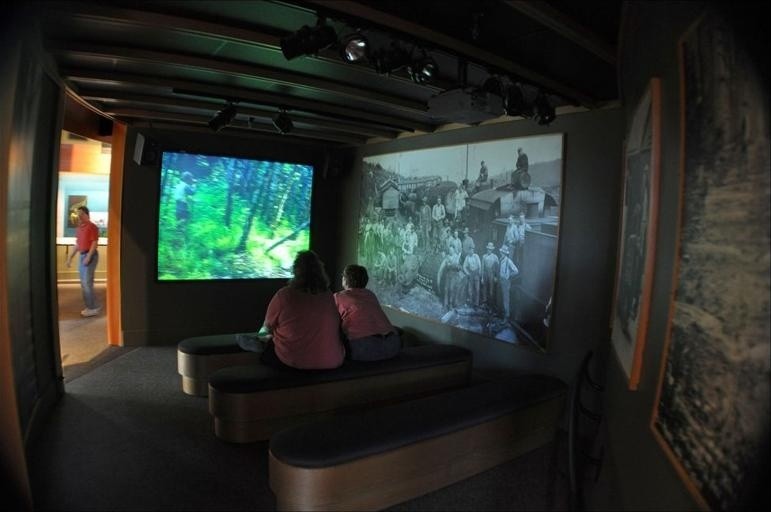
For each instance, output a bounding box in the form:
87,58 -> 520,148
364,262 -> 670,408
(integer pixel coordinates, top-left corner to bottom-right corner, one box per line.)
469,242 -> 509,255
462,227 -> 469,234
507,212 -> 526,220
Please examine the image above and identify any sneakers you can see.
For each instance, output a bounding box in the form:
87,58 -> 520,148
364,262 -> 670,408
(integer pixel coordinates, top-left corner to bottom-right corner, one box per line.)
81,307 -> 100,317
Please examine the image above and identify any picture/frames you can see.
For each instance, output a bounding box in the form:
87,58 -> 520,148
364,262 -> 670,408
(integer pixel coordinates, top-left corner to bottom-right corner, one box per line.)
650,0 -> 771,511
611,78 -> 661,391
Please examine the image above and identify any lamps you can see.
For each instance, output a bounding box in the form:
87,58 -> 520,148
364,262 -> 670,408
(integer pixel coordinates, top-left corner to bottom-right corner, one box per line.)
207,101 -> 296,139
281,9 -> 559,135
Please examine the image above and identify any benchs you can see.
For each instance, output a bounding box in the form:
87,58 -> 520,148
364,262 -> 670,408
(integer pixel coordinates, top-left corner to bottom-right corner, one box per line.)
269,374 -> 573,511
177,324 -> 405,397
208,344 -> 474,443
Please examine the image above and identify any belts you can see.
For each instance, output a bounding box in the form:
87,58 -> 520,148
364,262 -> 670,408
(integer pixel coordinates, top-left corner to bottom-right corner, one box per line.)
80,250 -> 88,254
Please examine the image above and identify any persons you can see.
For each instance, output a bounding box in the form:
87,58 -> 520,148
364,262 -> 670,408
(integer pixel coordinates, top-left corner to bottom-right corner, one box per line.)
358,148 -> 534,325
258,250 -> 346,378
174,171 -> 197,220
616,155 -> 650,333
331,263 -> 401,362
66,205 -> 102,318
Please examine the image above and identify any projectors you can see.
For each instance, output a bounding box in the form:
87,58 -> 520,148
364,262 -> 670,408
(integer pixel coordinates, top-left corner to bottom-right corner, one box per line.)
428,85 -> 504,125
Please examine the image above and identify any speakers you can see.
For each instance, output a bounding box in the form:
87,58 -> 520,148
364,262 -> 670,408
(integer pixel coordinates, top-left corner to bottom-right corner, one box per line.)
133,132 -> 160,167
322,150 -> 345,180
